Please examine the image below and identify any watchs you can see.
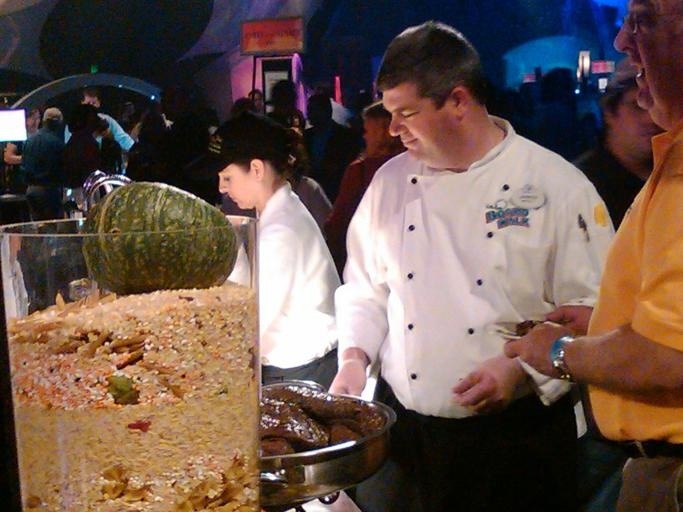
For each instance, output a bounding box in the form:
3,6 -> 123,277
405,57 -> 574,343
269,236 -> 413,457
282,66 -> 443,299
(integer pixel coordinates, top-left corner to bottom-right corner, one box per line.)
549,335 -> 575,386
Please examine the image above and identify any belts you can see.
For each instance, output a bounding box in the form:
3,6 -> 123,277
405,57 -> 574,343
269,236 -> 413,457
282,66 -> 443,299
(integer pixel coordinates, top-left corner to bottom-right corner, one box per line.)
622,439 -> 683,460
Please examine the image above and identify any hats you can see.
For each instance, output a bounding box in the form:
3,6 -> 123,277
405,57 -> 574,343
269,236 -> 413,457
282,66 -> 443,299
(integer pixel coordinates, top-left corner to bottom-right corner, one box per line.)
192,110 -> 291,180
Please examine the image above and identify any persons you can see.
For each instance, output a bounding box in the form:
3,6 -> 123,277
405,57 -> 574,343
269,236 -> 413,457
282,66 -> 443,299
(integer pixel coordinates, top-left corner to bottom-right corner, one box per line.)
1,77 -> 405,275
215,113 -> 342,392
504,1 -> 682,509
491,58 -> 667,228
326,20 -> 617,512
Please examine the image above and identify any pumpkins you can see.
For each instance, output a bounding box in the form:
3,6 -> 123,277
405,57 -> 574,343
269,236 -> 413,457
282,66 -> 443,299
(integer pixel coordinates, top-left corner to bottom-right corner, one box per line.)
80,181 -> 239,294
